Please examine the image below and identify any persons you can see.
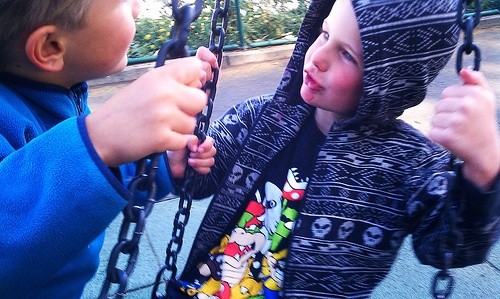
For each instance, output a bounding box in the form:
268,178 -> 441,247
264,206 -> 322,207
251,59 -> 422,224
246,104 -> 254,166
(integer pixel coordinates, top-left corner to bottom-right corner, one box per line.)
0,0 -> 217,299
166,0 -> 500,299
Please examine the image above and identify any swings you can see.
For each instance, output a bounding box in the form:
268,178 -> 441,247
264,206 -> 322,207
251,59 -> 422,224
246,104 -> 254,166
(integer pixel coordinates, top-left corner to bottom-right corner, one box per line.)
98,0 -> 482,299
